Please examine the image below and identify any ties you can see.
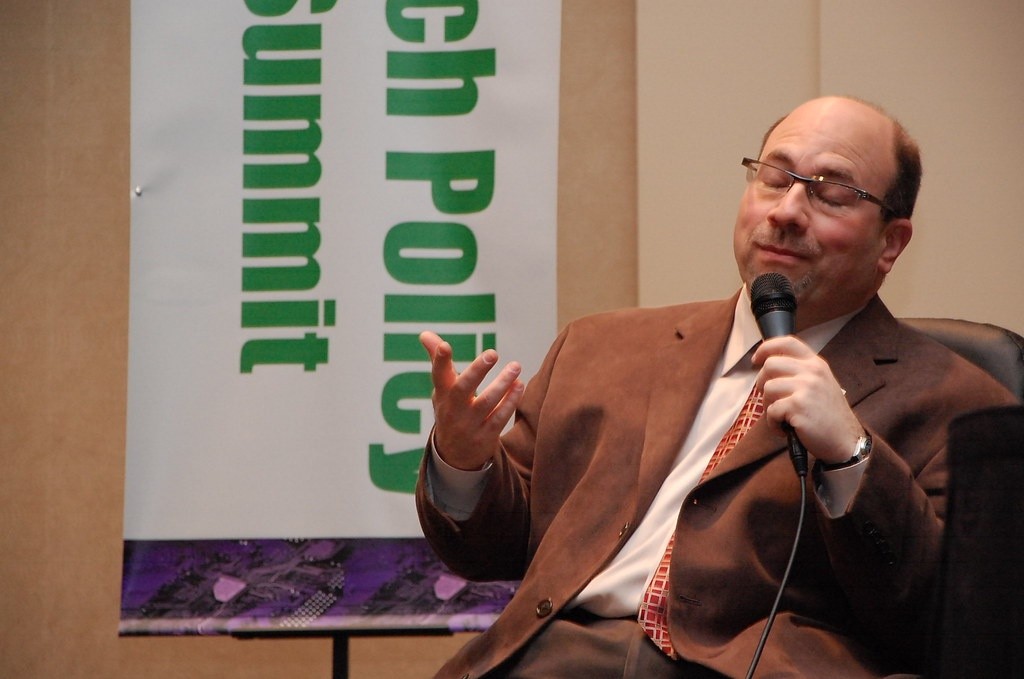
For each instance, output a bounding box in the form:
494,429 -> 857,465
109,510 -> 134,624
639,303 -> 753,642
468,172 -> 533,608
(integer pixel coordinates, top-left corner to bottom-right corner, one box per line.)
637,382 -> 768,660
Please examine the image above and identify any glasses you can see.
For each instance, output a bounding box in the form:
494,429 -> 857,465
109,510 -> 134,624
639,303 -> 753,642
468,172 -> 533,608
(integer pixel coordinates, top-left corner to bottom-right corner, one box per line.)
741,156 -> 894,217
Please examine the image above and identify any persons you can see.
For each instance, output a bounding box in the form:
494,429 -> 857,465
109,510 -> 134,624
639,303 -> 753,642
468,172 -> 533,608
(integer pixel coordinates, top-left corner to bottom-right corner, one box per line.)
416,95 -> 1024,679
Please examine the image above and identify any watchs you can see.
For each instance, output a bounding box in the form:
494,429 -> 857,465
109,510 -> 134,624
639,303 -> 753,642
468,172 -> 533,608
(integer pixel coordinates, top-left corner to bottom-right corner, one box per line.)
821,436 -> 872,470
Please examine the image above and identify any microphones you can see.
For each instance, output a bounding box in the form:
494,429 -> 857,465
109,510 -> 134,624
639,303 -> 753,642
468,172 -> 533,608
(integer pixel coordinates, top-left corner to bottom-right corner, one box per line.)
751,273 -> 809,479
